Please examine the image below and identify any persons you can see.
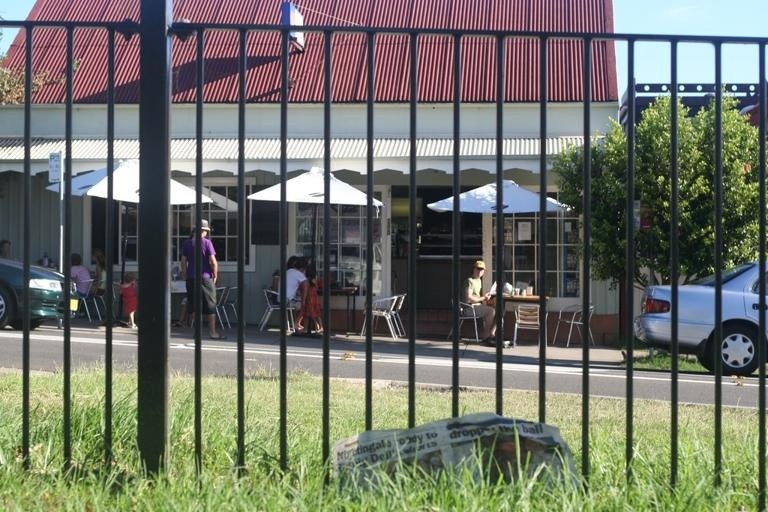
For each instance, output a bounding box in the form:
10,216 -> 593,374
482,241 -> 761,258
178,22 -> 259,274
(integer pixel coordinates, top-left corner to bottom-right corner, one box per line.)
463,262 -> 505,346
2,239 -> 139,327
182,219 -> 227,341
277,256 -> 325,335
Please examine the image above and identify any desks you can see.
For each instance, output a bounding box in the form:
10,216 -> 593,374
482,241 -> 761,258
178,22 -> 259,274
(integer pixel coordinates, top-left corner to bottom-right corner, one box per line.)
169,278 -> 190,296
313,287 -> 358,337
485,294 -> 549,348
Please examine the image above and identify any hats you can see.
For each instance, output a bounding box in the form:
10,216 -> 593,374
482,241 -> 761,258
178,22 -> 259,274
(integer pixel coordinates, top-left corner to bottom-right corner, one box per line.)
473,260 -> 486,270
192,218 -> 212,232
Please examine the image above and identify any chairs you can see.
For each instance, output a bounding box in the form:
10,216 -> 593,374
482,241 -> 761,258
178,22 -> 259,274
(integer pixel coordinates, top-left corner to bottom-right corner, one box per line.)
257,286 -> 297,335
358,293 -> 401,341
77,278 -> 107,324
221,286 -> 238,329
511,304 -> 547,350
445,297 -> 488,344
373,293 -> 409,337
189,286 -> 226,331
552,303 -> 595,348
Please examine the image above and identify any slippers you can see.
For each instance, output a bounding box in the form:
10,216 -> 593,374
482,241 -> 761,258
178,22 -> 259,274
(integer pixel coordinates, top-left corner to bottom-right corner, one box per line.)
209,332 -> 228,340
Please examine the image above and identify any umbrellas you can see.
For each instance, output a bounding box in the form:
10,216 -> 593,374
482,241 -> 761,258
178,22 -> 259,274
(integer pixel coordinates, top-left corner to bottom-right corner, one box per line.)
248,163 -> 383,271
186,185 -> 239,239
427,179 -> 576,295
43,159 -> 214,327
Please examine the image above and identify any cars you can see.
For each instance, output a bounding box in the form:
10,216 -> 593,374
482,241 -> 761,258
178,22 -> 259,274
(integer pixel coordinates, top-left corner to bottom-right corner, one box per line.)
310,241 -> 383,296
0,256 -> 79,331
634,262 -> 768,375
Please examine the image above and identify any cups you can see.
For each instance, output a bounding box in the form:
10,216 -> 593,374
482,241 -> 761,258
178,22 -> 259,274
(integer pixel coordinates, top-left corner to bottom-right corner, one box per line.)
511,285 -> 533,296
